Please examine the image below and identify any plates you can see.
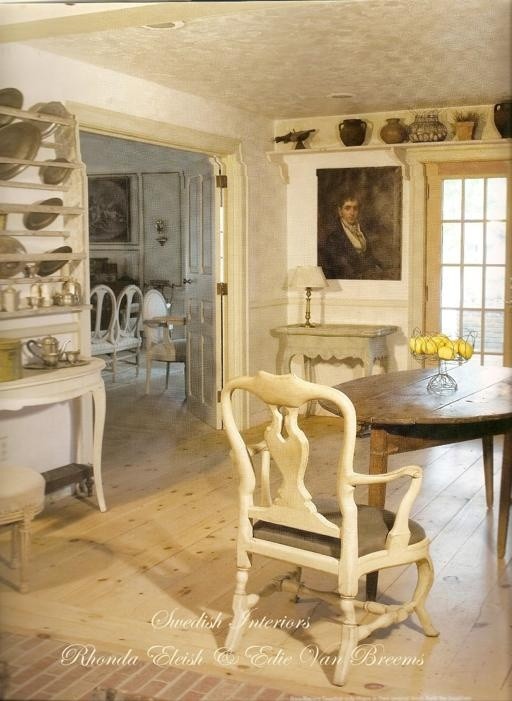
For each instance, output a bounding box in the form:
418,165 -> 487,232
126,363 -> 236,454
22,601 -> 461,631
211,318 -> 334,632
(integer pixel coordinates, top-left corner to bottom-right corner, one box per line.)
36,245 -> 73,278
0,235 -> 29,278
0,87 -> 26,125
0,120 -> 41,182
33,99 -> 65,140
42,156 -> 73,186
25,196 -> 65,232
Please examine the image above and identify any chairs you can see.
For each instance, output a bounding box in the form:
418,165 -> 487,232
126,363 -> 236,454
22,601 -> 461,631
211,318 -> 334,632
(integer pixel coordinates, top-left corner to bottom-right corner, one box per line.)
90,285 -> 186,401
220,371 -> 439,687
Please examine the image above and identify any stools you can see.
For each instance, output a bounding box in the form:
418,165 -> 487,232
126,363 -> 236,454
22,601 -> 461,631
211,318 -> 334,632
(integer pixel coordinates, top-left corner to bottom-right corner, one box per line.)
0,464 -> 46,595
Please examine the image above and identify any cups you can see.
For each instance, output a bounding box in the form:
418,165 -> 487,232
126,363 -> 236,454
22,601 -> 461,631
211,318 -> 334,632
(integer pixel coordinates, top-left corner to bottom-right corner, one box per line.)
65,350 -> 79,362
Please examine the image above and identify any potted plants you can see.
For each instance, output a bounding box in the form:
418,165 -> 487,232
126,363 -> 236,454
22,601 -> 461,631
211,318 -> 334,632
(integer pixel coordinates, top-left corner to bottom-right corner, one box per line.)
449,111 -> 480,141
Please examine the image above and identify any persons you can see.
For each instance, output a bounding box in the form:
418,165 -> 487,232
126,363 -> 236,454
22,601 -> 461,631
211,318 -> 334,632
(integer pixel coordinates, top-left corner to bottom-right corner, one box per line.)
322,192 -> 387,280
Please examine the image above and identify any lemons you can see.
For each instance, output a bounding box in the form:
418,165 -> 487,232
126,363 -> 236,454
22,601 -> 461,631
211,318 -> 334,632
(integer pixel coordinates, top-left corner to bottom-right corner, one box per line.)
438,345 -> 455,361
422,338 -> 437,355
409,335 -> 422,355
438,336 -> 454,349
454,337 -> 465,353
446,341 -> 460,354
459,341 -> 473,360
433,334 -> 444,346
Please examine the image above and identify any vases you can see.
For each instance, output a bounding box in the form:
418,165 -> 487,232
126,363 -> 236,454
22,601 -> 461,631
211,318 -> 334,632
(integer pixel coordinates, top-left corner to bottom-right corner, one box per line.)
494,103 -> 512,138
339,114 -> 447,146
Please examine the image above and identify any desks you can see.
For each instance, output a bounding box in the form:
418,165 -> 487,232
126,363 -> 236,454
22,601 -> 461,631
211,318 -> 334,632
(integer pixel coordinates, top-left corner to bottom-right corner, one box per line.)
271,323 -> 397,418
0,356 -> 108,513
318,365 -> 512,601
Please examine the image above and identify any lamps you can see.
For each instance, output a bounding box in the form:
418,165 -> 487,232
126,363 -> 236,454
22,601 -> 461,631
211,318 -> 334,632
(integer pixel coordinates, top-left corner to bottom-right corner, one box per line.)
156,219 -> 167,246
290,265 -> 329,328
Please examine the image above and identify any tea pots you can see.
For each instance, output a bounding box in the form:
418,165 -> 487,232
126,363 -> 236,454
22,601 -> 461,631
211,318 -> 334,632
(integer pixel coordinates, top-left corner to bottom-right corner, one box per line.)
26,333 -> 72,368
55,291 -> 79,306
2,284 -> 22,311
